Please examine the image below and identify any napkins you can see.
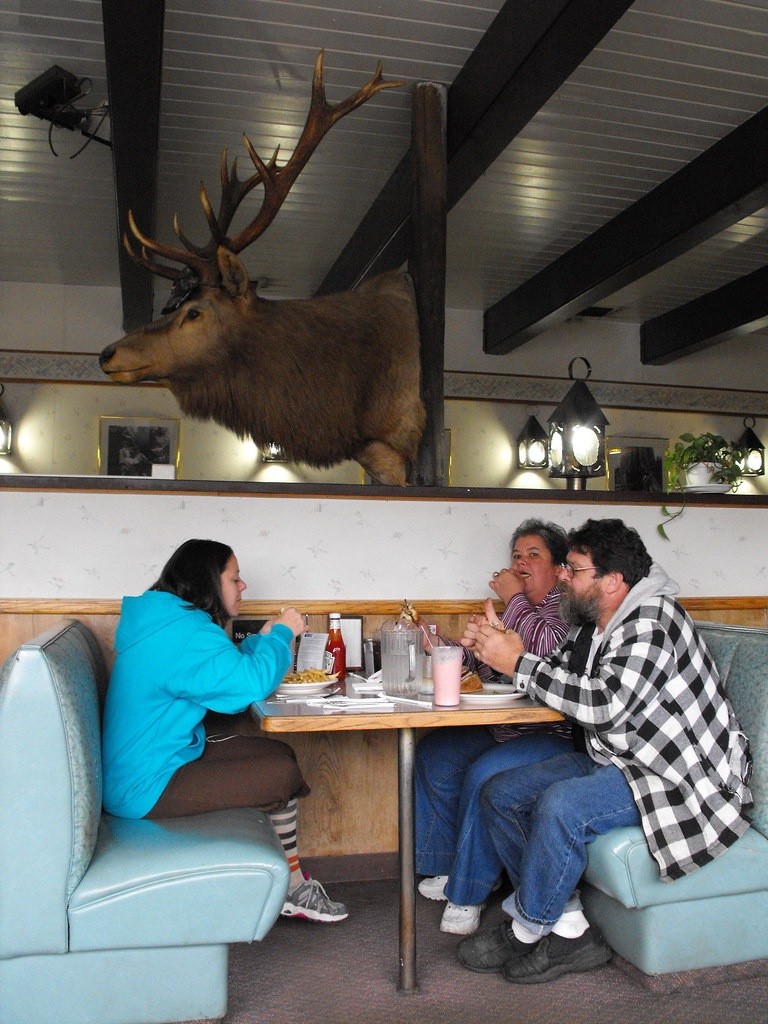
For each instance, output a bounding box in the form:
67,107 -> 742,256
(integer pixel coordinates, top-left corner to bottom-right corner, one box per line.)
351,681 -> 385,692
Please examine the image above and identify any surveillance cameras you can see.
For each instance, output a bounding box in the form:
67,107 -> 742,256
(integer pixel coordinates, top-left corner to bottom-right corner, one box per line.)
14,66 -> 83,115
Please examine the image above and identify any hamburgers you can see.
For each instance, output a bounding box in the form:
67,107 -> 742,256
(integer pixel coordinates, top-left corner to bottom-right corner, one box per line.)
398,598 -> 419,627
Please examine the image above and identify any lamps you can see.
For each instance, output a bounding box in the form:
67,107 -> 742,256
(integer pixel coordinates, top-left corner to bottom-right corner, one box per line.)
260,442 -> 290,463
0,381 -> 14,456
607,438 -> 622,455
515,404 -> 551,471
731,414 -> 766,477
545,356 -> 612,490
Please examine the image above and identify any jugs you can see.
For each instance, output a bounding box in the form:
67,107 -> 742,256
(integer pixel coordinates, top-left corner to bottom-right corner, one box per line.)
380,618 -> 424,697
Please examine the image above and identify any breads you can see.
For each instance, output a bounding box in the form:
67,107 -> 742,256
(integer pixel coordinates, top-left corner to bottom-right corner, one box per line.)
460,675 -> 484,694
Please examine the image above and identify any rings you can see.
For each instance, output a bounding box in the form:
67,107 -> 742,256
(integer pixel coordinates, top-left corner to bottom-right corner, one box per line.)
501,569 -> 507,572
493,572 -> 499,577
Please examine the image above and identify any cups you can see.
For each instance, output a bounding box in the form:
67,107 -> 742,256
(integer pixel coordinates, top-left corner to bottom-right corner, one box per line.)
432,646 -> 463,707
418,656 -> 435,695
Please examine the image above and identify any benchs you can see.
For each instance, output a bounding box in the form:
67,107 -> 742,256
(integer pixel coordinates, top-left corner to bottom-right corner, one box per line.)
576,619 -> 768,996
0,617 -> 291,1024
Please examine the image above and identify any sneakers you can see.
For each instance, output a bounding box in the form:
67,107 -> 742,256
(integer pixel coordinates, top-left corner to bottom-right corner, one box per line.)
455,918 -> 541,974
280,870 -> 349,924
439,900 -> 486,937
502,924 -> 613,984
418,871 -> 507,905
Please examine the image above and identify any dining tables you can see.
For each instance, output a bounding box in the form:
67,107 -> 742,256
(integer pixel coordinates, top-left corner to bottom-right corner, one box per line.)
249,670 -> 566,998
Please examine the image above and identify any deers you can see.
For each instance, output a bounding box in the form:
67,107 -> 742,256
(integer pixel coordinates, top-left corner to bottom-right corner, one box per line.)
98,46 -> 426,490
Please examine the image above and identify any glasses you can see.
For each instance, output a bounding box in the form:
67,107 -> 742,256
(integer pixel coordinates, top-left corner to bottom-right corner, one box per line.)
560,562 -> 601,578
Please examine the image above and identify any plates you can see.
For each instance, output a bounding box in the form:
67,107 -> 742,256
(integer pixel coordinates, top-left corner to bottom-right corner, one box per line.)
277,677 -> 339,695
458,683 -> 527,703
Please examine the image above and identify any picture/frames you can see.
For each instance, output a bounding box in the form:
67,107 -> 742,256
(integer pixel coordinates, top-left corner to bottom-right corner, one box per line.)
100,416 -> 183,478
605,435 -> 670,492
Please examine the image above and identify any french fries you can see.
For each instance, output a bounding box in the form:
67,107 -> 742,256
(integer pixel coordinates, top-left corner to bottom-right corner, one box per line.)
283,667 -> 342,684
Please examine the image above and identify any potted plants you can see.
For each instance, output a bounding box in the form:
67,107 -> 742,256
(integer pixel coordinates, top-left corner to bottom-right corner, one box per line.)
656,433 -> 747,543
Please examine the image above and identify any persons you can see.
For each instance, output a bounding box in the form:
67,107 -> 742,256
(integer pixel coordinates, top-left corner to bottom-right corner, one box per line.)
455,516 -> 756,984
411,518 -> 583,937
101,538 -> 350,924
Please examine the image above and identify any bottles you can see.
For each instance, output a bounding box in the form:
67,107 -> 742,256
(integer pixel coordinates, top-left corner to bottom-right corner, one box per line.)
326,612 -> 346,681
